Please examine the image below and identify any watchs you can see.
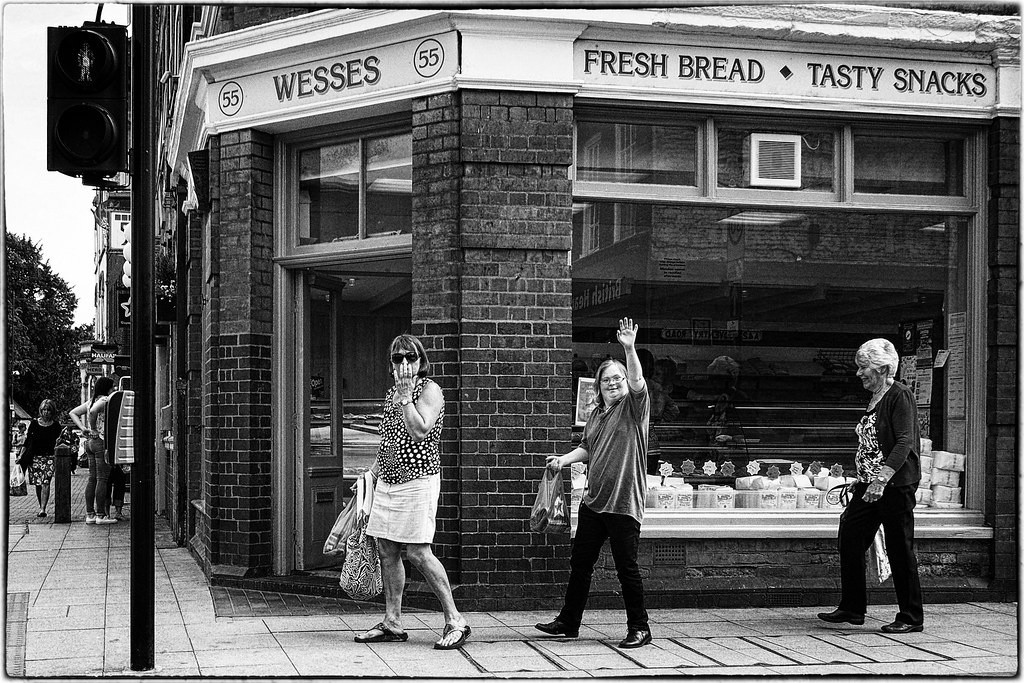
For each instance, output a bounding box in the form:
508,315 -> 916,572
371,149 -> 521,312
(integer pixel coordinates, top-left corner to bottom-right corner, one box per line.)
401,400 -> 413,405
876,476 -> 886,483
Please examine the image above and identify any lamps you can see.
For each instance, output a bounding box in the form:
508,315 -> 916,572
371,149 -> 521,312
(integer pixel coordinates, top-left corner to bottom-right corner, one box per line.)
159,72 -> 179,84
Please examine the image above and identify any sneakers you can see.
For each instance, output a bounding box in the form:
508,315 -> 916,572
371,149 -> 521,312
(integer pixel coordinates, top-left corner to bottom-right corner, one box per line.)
95,515 -> 117,524
86,515 -> 97,524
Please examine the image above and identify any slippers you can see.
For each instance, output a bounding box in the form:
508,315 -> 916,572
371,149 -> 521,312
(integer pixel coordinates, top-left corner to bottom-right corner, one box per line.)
434,625 -> 472,650
354,623 -> 408,643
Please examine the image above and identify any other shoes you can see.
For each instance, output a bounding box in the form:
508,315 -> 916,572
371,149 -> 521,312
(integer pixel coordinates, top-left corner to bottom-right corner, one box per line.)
37,512 -> 47,517
113,512 -> 127,521
107,515 -> 110,519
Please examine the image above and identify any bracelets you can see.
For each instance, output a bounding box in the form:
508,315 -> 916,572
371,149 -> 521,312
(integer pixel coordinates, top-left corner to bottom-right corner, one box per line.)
369,469 -> 377,479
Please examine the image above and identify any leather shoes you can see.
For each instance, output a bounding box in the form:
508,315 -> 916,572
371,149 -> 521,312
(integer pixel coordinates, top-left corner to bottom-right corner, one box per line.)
817,610 -> 865,625
535,618 -> 579,637
881,620 -> 924,633
619,628 -> 652,647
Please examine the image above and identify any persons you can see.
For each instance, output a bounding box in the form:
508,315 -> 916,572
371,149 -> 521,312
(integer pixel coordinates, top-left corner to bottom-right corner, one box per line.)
350,335 -> 471,650
12,423 -> 27,458
535,318 -> 654,648
22,399 -> 62,516
60,426 -> 79,474
706,356 -> 749,447
638,348 -> 693,424
68,378 -> 118,524
104,467 -> 127,521
817,338 -> 924,632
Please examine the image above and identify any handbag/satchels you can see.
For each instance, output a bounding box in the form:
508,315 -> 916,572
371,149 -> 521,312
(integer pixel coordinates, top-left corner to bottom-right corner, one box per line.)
323,472 -> 383,601
826,483 -> 892,588
9,464 -> 27,496
529,465 -> 570,534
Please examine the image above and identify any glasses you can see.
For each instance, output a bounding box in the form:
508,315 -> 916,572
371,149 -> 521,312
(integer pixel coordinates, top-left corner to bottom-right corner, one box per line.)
392,352 -> 422,364
600,375 -> 625,384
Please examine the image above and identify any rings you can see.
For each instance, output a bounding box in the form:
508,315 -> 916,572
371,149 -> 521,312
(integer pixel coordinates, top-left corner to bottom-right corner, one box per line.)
874,498 -> 877,501
